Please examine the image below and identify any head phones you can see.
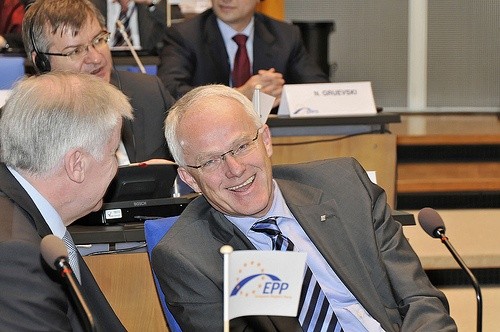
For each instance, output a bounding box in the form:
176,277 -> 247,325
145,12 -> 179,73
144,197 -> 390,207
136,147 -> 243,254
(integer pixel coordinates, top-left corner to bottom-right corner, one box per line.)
30,0 -> 51,73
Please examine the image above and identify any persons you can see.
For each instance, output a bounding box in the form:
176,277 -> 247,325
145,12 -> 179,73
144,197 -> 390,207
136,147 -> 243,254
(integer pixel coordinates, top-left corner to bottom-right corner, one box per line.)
21,0 -> 182,217
0,0 -> 33,55
152,83 -> 462,332
159,0 -> 330,119
85,0 -> 167,57
0,69 -> 137,332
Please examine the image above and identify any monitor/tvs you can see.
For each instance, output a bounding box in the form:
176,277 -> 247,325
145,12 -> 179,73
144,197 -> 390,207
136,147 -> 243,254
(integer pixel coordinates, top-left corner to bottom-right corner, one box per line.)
105,164 -> 178,202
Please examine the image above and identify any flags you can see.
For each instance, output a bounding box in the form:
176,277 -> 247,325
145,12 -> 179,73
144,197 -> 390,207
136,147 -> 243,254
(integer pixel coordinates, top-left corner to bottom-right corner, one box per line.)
227,249 -> 307,320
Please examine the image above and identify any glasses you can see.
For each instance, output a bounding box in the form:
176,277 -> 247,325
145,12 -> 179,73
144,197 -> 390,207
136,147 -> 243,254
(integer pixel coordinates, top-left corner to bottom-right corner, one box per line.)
38,31 -> 111,62
186,128 -> 259,174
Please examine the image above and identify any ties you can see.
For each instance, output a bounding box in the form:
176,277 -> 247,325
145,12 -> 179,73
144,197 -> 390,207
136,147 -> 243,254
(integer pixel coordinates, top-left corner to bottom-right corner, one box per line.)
62,231 -> 82,285
113,11 -> 133,46
232,34 -> 251,89
250,217 -> 345,332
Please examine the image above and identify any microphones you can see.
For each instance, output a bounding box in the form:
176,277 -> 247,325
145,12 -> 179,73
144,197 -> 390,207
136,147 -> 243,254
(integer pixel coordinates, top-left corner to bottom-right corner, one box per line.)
417,207 -> 483,332
40,234 -> 96,332
